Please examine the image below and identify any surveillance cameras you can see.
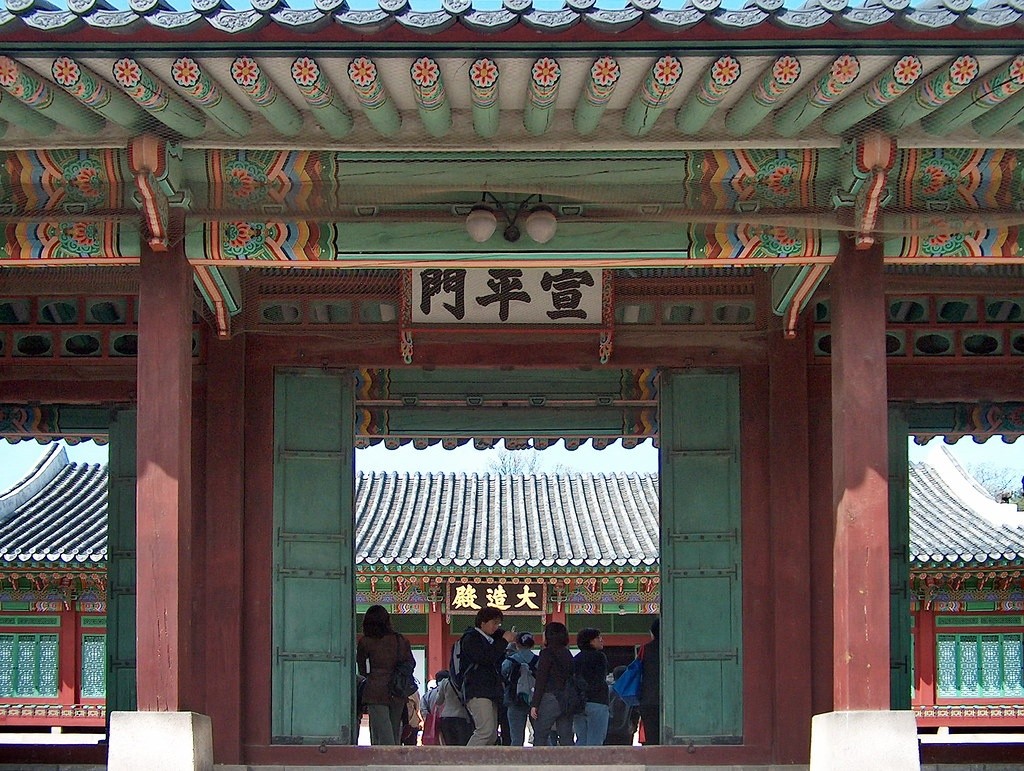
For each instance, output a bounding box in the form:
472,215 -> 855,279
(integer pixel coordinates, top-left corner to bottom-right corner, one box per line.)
505,226 -> 518,238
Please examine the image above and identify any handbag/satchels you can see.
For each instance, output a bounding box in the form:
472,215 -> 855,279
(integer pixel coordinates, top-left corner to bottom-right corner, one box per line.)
638,720 -> 646,743
558,677 -> 587,714
422,704 -> 446,745
388,632 -> 418,698
613,644 -> 644,706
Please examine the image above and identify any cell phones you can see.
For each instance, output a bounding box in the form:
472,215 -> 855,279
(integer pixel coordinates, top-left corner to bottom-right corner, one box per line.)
511,626 -> 516,633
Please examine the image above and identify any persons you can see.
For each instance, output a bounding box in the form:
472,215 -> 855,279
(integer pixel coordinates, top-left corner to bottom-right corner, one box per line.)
356,604 -> 660,746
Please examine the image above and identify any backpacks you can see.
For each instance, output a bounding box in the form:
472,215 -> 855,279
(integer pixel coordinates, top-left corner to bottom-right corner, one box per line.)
449,627 -> 487,692
505,654 -> 540,705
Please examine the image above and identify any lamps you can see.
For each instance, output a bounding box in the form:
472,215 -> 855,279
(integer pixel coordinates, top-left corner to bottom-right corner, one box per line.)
466,181 -> 558,243
